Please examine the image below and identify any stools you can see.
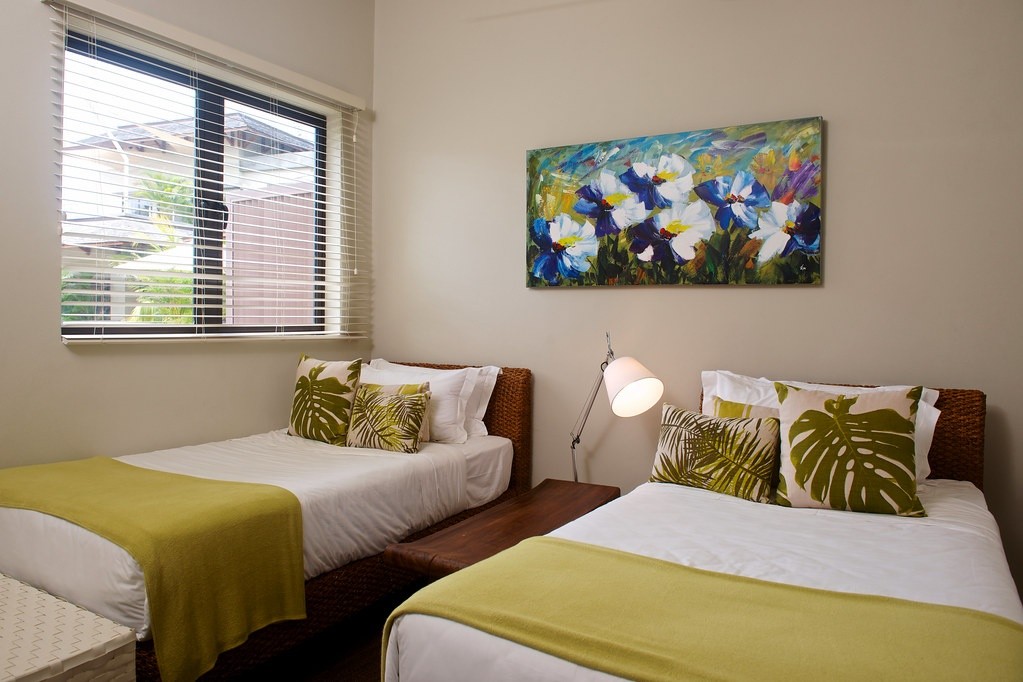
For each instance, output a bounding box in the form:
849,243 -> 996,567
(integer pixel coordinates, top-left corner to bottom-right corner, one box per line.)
386,479 -> 623,584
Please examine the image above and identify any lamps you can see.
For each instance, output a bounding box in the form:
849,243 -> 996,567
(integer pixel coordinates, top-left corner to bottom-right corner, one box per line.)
570,333 -> 664,482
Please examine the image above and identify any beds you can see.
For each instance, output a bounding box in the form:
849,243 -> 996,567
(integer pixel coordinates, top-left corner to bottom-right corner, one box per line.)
378,387 -> 1023,682
0,366 -> 533,682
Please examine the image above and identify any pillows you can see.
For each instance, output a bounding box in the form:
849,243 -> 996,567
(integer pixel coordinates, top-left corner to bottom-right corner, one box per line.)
286,354 -> 363,447
701,368 -> 942,494
774,381 -> 928,517
370,358 -> 504,438
647,403 -> 779,505
360,363 -> 483,444
360,382 -> 433,442
345,386 -> 432,454
713,395 -> 779,419
